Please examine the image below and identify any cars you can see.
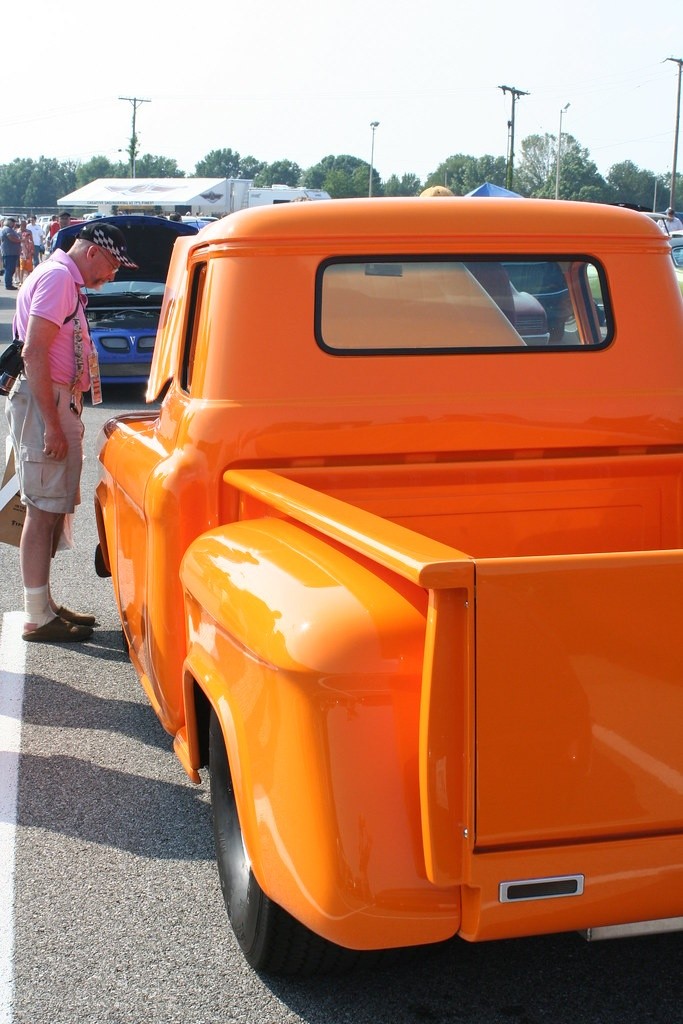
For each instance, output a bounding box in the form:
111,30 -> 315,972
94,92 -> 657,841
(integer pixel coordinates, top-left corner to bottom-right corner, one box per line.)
44,212 -> 200,400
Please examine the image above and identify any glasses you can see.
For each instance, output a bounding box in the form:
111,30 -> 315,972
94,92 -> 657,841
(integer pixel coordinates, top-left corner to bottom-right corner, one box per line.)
87,244 -> 119,274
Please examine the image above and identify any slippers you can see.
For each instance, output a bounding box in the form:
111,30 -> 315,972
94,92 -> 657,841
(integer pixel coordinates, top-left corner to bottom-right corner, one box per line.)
22,616 -> 93,642
57,607 -> 95,625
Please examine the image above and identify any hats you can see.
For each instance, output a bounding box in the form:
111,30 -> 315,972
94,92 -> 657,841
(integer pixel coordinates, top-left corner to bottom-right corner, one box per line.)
664,207 -> 674,214
7,217 -> 19,222
58,211 -> 70,218
419,185 -> 453,196
75,222 -> 140,271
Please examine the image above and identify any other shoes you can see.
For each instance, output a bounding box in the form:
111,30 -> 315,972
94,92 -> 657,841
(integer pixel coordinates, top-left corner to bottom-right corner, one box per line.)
6,286 -> 17,289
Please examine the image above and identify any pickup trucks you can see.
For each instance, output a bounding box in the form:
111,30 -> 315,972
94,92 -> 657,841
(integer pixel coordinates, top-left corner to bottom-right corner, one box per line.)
86,192 -> 683,973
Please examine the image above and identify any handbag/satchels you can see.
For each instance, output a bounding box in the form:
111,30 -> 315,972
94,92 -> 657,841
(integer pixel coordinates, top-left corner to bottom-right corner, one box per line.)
0,337 -> 27,395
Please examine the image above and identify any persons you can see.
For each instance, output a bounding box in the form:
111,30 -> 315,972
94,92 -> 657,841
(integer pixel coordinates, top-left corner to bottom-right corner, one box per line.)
656,207 -> 683,237
0,208 -> 208,290
4,221 -> 139,642
